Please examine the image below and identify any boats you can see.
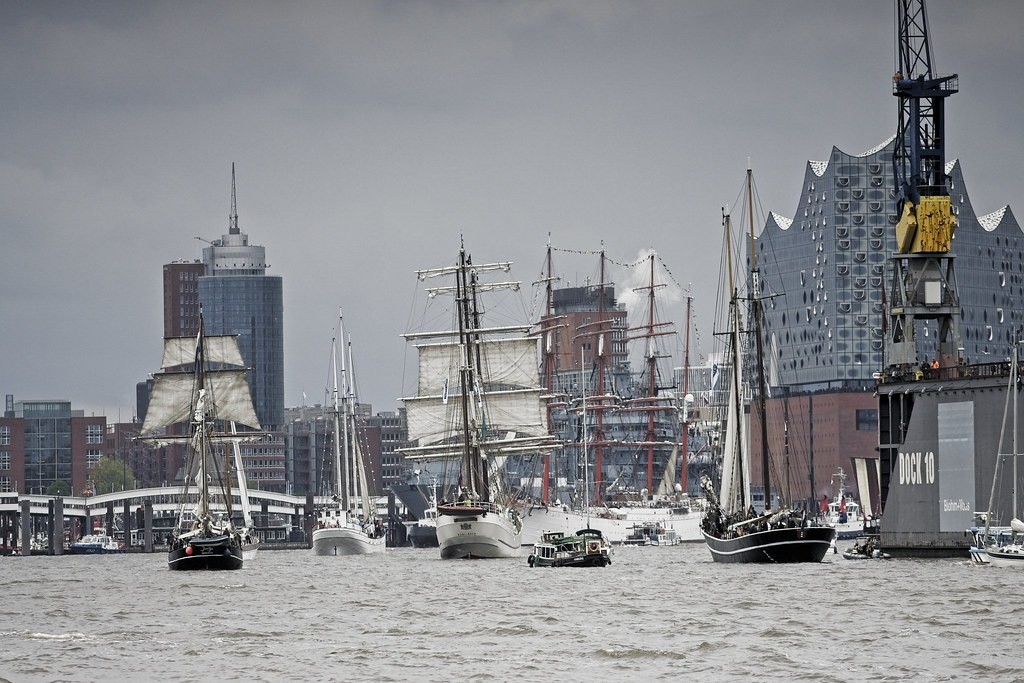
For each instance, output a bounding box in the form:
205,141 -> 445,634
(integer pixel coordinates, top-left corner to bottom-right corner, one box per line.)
842,536 -> 893,560
819,466 -> 872,538
69,532 -> 126,554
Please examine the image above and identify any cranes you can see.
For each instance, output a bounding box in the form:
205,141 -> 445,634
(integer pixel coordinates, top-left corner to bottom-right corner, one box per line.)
878,0 -> 976,386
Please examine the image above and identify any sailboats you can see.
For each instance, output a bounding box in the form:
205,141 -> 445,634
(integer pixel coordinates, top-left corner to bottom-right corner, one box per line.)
956,323 -> 1024,568
393,226 -> 695,570
167,303 -> 261,573
695,155 -> 839,569
303,304 -> 388,557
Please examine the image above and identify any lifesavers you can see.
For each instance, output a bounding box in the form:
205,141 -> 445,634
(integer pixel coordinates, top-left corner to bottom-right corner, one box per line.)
551,561 -> 555,566
593,559 -> 601,566
528,555 -> 533,567
670,497 -> 674,501
589,543 -> 598,552
